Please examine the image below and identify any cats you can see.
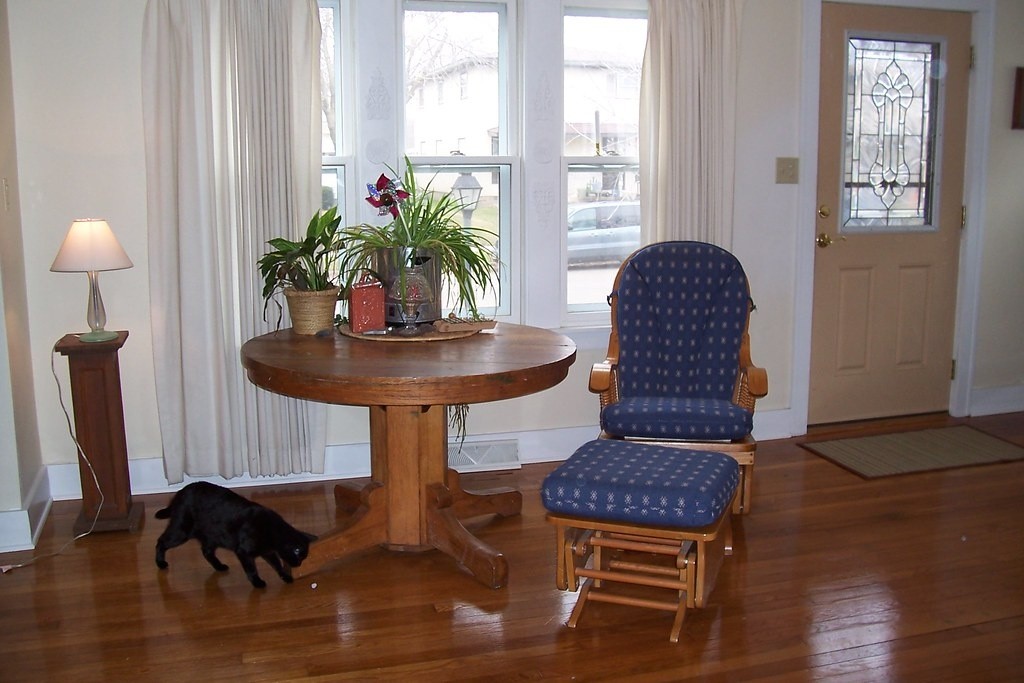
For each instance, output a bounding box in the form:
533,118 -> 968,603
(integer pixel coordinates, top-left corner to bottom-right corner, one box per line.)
155,481 -> 319,589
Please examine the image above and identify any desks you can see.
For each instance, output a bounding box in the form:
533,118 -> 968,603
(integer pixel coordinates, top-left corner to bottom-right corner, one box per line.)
241,319 -> 577,591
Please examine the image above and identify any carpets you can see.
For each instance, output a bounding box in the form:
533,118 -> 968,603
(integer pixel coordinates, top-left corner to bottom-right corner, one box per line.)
796,422 -> 1024,480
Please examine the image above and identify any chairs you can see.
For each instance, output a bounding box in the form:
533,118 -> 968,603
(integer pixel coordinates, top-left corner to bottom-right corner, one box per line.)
589,241 -> 767,514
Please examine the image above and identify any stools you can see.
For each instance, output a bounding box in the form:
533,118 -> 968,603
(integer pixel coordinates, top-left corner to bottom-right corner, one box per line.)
543,439 -> 741,642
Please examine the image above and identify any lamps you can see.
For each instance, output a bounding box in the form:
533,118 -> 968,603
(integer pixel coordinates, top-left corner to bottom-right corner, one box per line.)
452,174 -> 483,215
49,219 -> 134,343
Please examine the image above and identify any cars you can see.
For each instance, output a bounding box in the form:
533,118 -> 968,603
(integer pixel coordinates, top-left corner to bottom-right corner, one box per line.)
567,200 -> 641,264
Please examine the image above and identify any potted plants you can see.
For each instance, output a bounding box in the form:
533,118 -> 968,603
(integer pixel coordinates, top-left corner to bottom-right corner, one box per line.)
255,205 -> 348,336
327,154 -> 507,322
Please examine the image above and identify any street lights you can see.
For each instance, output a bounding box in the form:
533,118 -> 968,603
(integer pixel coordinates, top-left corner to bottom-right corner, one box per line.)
451,173 -> 483,308
